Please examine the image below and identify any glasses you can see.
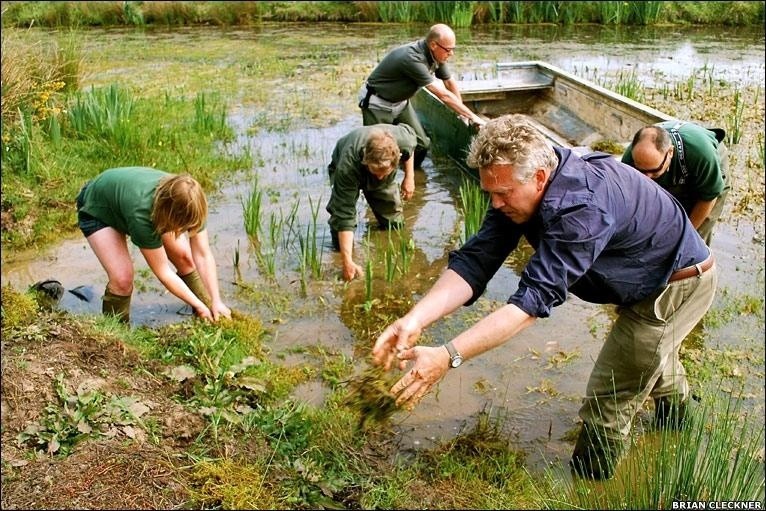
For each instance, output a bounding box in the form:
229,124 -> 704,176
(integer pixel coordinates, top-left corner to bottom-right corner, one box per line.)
634,153 -> 670,175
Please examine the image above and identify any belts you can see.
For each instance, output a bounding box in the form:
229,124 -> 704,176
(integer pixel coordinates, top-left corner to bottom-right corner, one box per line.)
667,244 -> 717,283
363,81 -> 408,104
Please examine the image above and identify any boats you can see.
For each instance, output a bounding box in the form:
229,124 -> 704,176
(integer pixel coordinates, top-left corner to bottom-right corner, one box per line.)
405,53 -> 676,196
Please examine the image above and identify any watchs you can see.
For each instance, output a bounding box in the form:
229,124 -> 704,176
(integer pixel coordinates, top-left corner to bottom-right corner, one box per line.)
445,340 -> 464,370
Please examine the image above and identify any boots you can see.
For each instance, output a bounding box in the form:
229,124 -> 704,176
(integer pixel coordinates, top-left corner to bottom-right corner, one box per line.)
567,420 -> 629,482
176,268 -> 241,318
651,397 -> 693,433
398,144 -> 430,171
330,226 -> 355,252
102,283 -> 134,327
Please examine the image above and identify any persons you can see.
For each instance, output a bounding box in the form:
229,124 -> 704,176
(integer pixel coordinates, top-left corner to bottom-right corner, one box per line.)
358,23 -> 475,171
369,112 -> 720,483
73,167 -> 232,323
327,121 -> 416,279
621,118 -> 729,244
